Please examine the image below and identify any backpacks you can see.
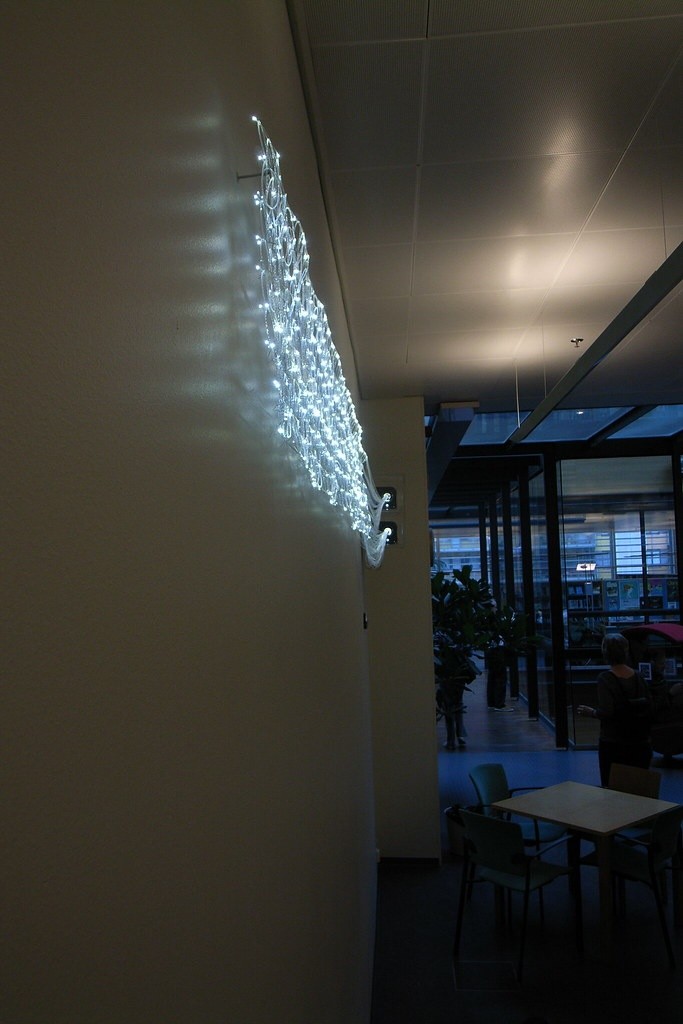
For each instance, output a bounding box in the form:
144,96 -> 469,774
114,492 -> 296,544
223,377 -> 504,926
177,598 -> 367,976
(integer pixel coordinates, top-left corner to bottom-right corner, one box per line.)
609,668 -> 652,743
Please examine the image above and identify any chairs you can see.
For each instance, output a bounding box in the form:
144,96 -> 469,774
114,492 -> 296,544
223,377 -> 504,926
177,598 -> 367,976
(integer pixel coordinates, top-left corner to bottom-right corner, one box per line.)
453,762 -> 681,989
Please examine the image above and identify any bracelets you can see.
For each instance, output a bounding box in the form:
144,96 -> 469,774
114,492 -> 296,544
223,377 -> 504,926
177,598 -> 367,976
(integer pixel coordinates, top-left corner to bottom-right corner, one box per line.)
591,709 -> 596,718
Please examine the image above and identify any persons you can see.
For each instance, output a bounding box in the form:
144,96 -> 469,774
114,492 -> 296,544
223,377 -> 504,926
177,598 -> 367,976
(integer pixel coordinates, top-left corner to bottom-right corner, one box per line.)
443,693 -> 470,751
577,633 -> 654,787
482,644 -> 512,711
642,649 -> 683,725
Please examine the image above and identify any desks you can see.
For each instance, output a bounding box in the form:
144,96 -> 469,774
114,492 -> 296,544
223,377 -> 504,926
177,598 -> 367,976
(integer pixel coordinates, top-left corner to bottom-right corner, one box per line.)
490,779 -> 681,974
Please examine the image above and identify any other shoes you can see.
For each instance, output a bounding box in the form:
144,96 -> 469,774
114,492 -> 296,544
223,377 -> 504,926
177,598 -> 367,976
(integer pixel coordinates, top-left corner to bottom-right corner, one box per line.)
488,707 -> 494,711
494,707 -> 514,712
459,738 -> 466,745
444,741 -> 455,749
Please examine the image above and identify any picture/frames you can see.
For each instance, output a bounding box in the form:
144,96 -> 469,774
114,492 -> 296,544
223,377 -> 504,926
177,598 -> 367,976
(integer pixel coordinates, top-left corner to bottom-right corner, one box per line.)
638,662 -> 652,680
663,659 -> 676,676
619,580 -> 640,608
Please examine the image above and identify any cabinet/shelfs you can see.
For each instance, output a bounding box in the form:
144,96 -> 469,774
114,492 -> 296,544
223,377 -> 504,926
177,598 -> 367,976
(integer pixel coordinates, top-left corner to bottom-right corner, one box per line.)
514,579 -> 605,626
